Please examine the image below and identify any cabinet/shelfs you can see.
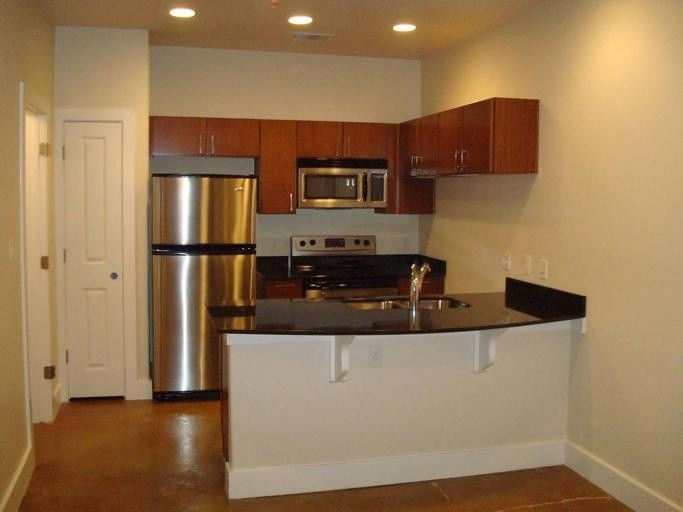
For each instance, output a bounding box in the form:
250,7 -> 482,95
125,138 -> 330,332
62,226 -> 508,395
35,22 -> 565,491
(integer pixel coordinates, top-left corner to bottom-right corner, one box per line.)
375,123 -> 436,214
399,113 -> 438,179
261,279 -> 302,299
257,120 -> 297,215
398,275 -> 443,294
297,120 -> 389,162
150,116 -> 259,157
438,96 -> 540,176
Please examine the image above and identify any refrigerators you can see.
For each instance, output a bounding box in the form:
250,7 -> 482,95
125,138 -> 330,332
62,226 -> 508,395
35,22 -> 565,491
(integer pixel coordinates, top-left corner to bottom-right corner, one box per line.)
152,173 -> 257,401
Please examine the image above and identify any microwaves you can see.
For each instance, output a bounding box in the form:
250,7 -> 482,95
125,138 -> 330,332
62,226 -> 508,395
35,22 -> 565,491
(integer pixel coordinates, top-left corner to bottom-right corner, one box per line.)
297,159 -> 387,208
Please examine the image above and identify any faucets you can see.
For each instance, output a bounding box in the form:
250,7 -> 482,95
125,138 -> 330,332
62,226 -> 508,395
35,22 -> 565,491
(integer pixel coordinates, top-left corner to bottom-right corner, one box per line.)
409,263 -> 431,309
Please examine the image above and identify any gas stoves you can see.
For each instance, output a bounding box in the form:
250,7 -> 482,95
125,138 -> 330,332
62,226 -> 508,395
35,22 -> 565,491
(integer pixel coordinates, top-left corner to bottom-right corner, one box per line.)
290,235 -> 398,289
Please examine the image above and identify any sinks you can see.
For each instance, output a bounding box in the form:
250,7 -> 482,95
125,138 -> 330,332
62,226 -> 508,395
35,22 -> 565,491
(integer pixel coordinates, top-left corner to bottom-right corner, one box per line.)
343,295 -> 475,312
